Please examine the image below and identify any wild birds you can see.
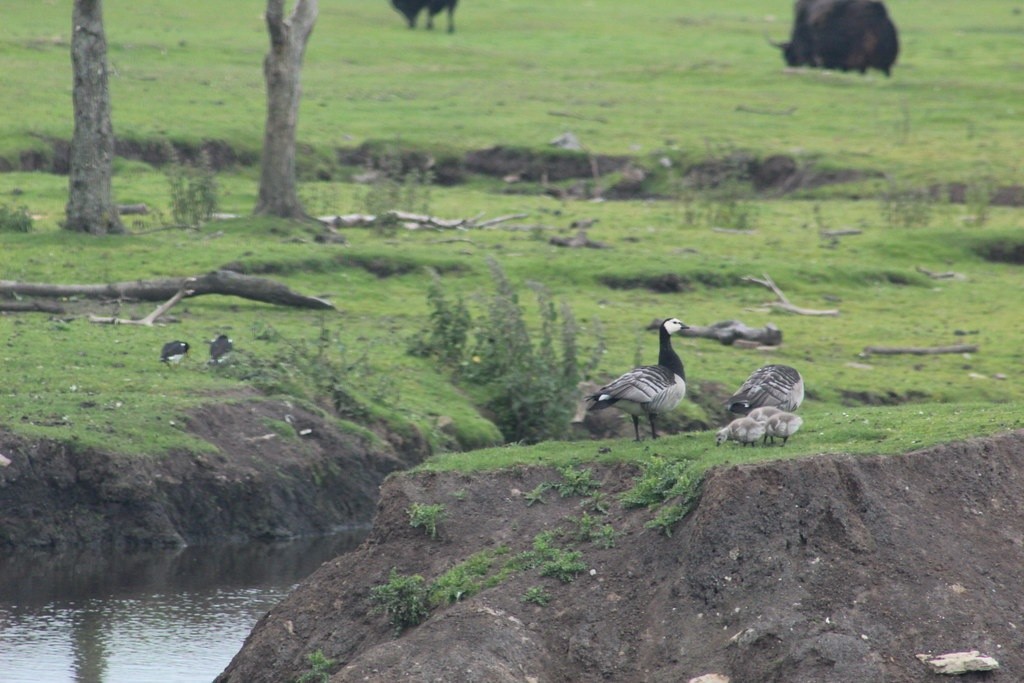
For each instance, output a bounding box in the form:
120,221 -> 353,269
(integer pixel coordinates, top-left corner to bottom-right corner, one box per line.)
746,406 -> 803,447
584,317 -> 689,442
210,335 -> 232,364
159,340 -> 189,370
723,365 -> 805,416
716,418 -> 766,447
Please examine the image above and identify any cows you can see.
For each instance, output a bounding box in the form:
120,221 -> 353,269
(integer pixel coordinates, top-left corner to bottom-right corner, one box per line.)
391,0 -> 458,32
766,0 -> 898,77
